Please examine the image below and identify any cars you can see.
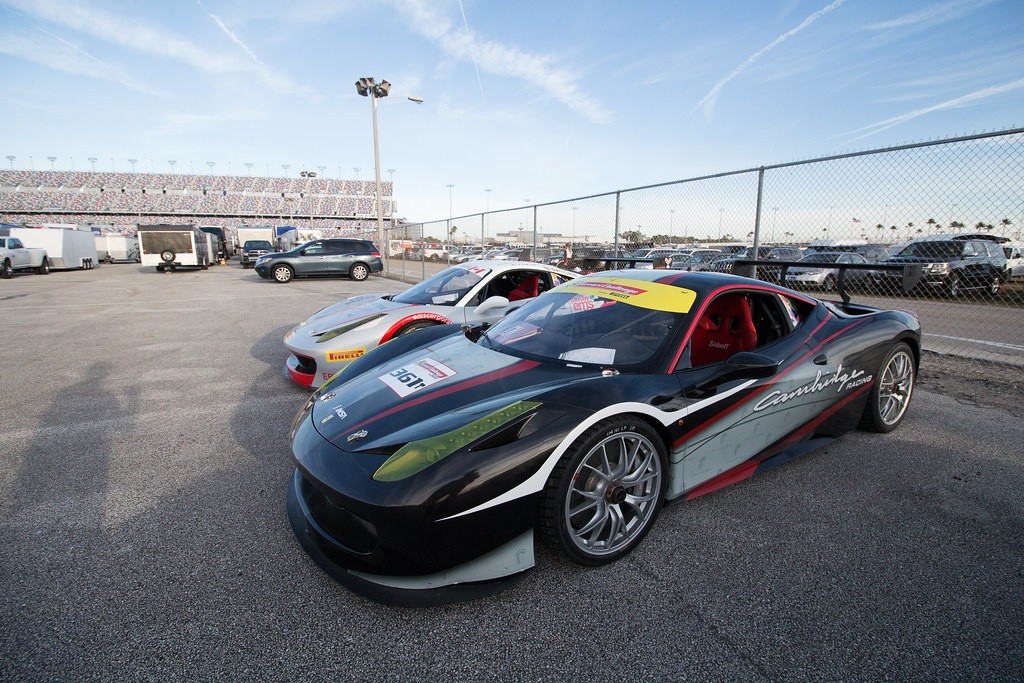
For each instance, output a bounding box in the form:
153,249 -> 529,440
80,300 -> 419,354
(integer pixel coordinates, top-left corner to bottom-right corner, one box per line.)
866,240 -> 1008,299
777,252 -> 872,293
387,236 -> 802,282
1001,245 -> 1024,279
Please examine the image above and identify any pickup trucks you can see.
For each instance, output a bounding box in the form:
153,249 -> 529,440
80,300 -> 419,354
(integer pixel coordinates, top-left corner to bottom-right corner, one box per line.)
0,236 -> 52,279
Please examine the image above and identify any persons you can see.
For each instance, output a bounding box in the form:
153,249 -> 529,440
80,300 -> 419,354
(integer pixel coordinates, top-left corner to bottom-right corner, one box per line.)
564,243 -> 574,270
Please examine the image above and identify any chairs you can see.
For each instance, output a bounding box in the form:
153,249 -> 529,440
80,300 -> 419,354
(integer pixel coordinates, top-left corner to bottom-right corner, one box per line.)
690,295 -> 758,367
508,273 -> 539,302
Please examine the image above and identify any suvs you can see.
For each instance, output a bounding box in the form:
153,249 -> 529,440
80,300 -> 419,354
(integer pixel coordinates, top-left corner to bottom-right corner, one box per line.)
241,240 -> 277,270
254,238 -> 384,283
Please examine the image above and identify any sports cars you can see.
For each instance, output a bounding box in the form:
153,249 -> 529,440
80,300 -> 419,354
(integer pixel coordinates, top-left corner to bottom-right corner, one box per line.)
285,268 -> 923,609
280,259 -> 611,392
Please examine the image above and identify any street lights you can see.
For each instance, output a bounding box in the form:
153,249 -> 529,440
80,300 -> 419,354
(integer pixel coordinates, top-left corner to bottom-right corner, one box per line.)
446,184 -> 455,244
354,76 -> 423,280
300,171 -> 319,229
